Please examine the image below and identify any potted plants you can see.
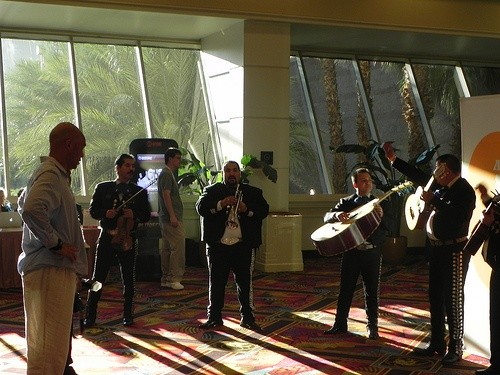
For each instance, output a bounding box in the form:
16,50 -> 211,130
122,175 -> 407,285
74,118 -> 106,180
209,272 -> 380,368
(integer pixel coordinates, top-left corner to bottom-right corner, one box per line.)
335,142 -> 440,266
171,143 -> 278,273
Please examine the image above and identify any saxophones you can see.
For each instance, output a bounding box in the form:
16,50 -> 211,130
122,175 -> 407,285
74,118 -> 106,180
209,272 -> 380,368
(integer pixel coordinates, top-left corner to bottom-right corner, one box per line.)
220,184 -> 244,245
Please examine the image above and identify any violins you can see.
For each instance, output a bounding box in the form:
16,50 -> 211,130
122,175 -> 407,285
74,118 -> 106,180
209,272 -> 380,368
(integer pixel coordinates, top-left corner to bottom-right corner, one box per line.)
111,199 -> 134,244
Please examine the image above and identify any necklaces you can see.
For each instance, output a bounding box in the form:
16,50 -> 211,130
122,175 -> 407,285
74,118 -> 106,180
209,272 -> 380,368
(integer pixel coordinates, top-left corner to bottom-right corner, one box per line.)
164,164 -> 178,188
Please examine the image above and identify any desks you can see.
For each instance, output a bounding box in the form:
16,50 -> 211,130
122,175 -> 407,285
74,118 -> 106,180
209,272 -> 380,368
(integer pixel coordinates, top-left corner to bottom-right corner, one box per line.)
0,228 -> 101,289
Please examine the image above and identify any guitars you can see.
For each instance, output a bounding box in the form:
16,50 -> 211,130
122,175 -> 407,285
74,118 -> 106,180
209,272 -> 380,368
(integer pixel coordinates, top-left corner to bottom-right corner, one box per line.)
310,178 -> 414,256
463,190 -> 500,256
405,163 -> 450,230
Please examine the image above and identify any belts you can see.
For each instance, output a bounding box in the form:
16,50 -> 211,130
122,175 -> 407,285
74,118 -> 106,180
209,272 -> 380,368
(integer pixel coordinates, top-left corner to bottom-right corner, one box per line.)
428,236 -> 466,246
359,243 -> 372,251
106,230 -> 118,235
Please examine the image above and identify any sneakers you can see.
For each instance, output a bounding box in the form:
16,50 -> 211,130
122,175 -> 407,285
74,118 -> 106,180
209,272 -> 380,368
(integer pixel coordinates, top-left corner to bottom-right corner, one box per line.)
165,281 -> 184,289
160,282 -> 166,286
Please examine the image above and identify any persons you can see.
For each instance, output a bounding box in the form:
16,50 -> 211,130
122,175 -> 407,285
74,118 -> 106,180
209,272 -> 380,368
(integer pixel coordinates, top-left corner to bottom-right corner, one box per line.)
195,161 -> 270,332
323,168 -> 395,339
83,153 -> 151,328
0,190 -> 12,212
383,142 -> 476,365
158,147 -> 185,290
13,189 -> 23,212
17,122 -> 89,375
474,183 -> 500,375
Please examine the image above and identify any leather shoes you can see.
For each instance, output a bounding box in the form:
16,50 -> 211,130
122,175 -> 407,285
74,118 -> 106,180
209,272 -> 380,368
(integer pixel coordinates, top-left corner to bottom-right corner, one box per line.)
475,364 -> 500,375
414,343 -> 446,355
441,354 -> 462,365
122,318 -> 133,326
240,321 -> 261,331
324,326 -> 347,334
199,319 -> 223,328
83,319 -> 95,325
369,333 -> 378,339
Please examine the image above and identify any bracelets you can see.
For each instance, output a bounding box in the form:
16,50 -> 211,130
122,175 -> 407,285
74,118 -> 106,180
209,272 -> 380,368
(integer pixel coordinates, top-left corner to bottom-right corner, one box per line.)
49,238 -> 63,251
